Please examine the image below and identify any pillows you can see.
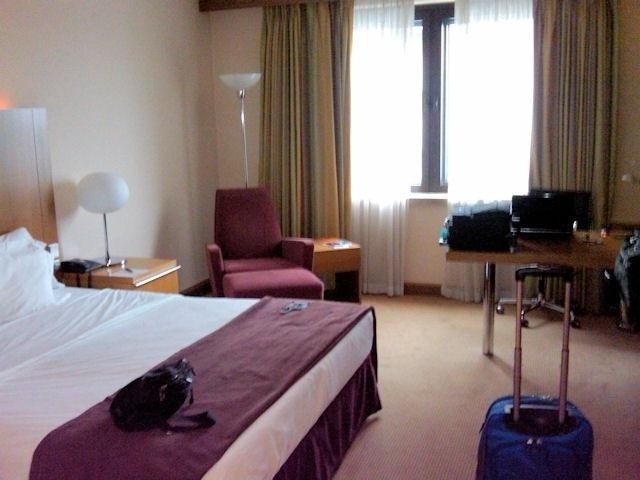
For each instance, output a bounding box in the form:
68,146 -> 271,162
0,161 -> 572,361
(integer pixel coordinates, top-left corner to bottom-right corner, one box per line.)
2,251 -> 69,325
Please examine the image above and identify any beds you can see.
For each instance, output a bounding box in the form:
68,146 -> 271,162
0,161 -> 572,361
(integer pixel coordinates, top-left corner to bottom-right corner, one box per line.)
1,106 -> 381,480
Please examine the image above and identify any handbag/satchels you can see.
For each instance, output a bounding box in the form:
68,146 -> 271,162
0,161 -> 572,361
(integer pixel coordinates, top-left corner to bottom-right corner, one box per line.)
109,357 -> 212,435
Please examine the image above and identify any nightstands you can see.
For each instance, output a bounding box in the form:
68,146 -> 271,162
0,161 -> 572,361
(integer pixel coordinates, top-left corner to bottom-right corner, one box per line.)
60,255 -> 180,293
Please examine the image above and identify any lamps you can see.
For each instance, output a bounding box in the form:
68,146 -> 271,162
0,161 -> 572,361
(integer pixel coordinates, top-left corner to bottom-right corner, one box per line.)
220,74 -> 262,189
76,172 -> 129,268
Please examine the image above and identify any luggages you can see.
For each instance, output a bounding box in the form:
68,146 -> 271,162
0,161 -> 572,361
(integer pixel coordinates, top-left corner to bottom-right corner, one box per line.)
475,264 -> 593,475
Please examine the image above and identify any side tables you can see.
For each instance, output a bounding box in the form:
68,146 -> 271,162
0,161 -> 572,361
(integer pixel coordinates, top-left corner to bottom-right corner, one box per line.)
307,234 -> 362,303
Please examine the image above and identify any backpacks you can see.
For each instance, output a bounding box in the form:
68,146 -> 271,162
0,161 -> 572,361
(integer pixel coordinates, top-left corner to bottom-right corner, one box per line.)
446,210 -> 521,253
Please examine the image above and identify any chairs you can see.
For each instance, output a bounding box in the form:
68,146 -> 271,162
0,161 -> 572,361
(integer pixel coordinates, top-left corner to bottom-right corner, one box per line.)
204,188 -> 323,299
497,188 -> 590,327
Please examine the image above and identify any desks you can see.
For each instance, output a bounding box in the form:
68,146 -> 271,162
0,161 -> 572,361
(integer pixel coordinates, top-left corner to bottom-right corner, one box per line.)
447,231 -> 639,356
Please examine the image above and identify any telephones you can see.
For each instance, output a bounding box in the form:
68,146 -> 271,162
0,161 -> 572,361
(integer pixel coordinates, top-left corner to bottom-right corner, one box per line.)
60,258 -> 102,273
